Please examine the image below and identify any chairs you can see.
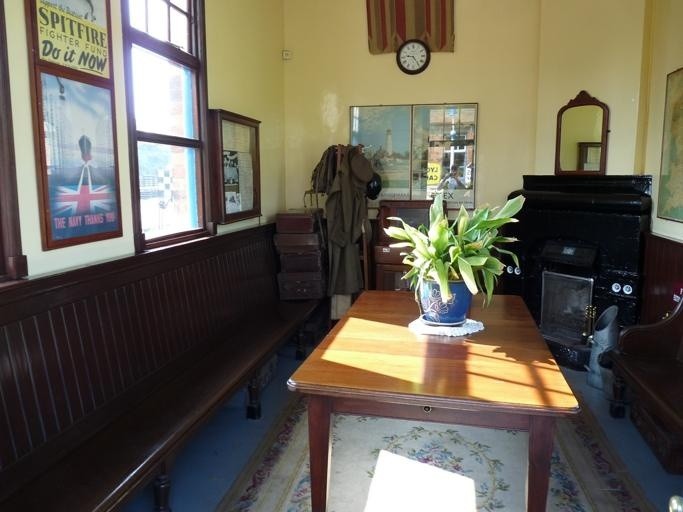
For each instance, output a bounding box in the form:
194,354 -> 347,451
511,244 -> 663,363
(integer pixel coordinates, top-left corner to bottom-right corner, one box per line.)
374,198 -> 448,289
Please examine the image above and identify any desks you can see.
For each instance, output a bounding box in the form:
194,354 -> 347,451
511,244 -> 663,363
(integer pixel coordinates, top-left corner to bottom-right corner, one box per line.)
286,289 -> 582,512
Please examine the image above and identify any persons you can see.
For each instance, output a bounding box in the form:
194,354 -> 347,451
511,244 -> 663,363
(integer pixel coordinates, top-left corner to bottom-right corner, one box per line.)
436,164 -> 466,189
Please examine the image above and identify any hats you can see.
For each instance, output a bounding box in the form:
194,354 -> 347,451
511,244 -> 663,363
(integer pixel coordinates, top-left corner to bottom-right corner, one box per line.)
350,156 -> 373,188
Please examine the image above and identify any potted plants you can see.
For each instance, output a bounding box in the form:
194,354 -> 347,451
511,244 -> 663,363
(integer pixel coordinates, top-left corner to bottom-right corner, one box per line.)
383,188 -> 526,327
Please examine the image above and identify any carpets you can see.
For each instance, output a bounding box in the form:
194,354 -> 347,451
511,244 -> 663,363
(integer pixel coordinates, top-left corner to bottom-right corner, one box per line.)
215,390 -> 660,511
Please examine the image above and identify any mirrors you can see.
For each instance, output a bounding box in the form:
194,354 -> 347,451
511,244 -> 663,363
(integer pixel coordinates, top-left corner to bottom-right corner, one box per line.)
556,89 -> 610,175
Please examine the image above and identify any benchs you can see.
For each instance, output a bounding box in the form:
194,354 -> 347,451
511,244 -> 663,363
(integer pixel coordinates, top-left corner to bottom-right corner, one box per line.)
0,221 -> 323,512
608,296 -> 683,475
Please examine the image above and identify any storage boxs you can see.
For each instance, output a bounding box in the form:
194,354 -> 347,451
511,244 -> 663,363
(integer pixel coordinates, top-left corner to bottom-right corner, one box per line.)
270,205 -> 330,301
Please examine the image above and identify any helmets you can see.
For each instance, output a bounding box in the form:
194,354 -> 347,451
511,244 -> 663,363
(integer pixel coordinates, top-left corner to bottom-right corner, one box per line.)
367,174 -> 382,200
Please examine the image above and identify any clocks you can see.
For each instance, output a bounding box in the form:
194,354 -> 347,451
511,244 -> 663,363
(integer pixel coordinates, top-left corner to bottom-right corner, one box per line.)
396,39 -> 430,75
